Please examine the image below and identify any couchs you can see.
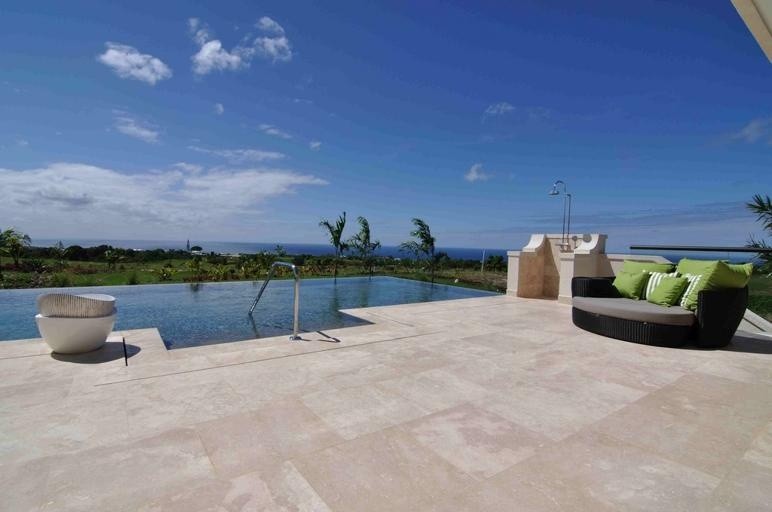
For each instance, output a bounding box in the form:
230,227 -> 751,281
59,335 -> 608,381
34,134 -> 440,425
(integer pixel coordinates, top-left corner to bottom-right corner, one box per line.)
571,275 -> 749,350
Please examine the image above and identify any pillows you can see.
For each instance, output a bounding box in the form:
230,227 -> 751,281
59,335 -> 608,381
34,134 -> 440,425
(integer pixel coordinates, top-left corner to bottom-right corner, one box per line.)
612,257 -> 753,310
